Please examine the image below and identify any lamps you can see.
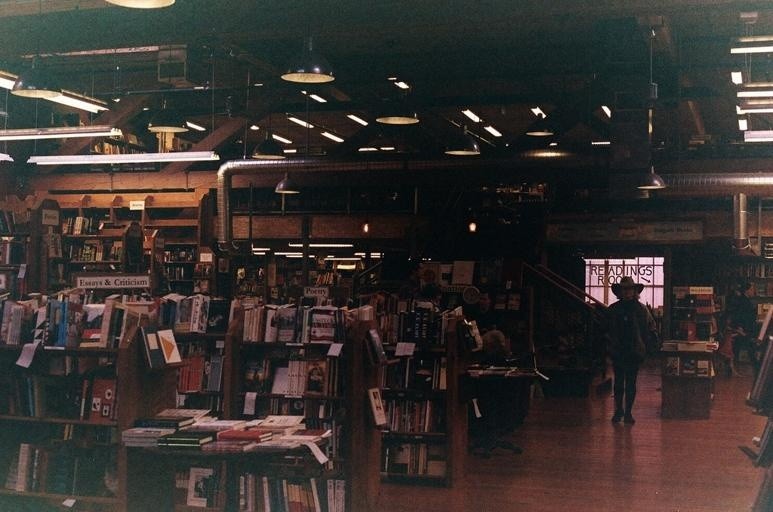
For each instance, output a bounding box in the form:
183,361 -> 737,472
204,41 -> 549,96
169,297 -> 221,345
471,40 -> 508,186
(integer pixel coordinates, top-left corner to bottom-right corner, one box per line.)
1,0 -> 224,173
250,101 -> 286,161
636,107 -> 669,191
444,120 -> 483,157
278,23 -> 337,85
375,80 -> 421,126
524,112 -> 556,138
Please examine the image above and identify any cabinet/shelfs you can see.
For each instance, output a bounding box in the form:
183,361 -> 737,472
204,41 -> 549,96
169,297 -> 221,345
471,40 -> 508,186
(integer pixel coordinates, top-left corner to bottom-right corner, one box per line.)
662,237 -> 773,420
0,194 -> 534,512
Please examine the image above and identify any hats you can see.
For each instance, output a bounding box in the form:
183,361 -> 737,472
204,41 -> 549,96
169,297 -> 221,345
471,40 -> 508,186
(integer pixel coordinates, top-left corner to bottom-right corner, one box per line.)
610,275 -> 644,300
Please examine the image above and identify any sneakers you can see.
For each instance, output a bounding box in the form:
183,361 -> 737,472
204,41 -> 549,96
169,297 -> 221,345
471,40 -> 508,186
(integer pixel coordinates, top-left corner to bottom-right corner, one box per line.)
610,406 -> 635,424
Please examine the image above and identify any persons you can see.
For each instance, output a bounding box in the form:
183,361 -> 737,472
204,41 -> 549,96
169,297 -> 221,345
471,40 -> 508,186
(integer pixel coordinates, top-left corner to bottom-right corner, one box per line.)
730,282 -> 760,376
591,276 -> 659,425
463,286 -> 499,334
458,330 -> 519,436
718,313 -> 743,377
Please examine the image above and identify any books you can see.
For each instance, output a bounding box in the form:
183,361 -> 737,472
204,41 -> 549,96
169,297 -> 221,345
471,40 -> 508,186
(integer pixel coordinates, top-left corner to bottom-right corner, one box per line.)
745,334 -> 772,512
661,285 -> 719,379
699,261 -> 773,335
452,255 -> 522,314
0,193 -> 453,512
458,320 -> 482,354
